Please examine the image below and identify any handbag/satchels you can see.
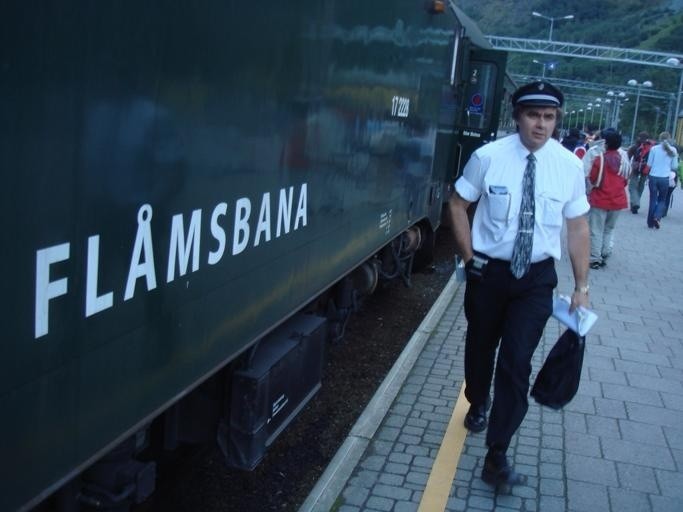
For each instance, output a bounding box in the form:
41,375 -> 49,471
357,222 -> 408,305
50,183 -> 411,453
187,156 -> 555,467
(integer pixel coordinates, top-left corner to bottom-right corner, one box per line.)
530,328 -> 586,411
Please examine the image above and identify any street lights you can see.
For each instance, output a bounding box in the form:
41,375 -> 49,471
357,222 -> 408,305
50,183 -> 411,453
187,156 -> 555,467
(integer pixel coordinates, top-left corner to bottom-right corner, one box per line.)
562,79 -> 652,146
667,58 -> 682,139
526,11 -> 574,82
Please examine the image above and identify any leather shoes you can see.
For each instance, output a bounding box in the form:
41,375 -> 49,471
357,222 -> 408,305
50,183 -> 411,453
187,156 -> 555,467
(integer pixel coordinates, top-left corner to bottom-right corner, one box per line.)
481,456 -> 527,486
464,394 -> 491,432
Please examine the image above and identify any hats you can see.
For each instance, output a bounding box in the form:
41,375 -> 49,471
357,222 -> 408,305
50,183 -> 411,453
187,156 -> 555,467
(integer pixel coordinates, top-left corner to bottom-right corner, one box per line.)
512,81 -> 564,106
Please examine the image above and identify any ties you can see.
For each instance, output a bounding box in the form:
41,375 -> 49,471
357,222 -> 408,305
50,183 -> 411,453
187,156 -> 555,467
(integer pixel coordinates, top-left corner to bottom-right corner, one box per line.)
509,153 -> 537,280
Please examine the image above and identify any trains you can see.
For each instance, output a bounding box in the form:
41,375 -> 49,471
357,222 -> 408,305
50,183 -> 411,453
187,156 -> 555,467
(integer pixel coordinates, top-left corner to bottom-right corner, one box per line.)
0,1 -> 518,509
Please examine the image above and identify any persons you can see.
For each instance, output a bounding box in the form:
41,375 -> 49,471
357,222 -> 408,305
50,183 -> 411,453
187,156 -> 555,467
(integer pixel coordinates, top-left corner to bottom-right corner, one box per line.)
448,80 -> 592,487
550,108 -> 678,270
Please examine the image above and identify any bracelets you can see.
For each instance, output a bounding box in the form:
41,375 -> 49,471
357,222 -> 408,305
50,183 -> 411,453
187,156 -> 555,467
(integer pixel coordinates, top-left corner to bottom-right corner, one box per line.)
572,285 -> 589,294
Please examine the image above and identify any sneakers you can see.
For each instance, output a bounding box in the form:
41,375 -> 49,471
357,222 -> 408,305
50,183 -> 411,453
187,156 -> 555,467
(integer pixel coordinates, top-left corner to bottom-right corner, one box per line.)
648,217 -> 659,228
589,254 -> 607,269
631,205 -> 640,214
